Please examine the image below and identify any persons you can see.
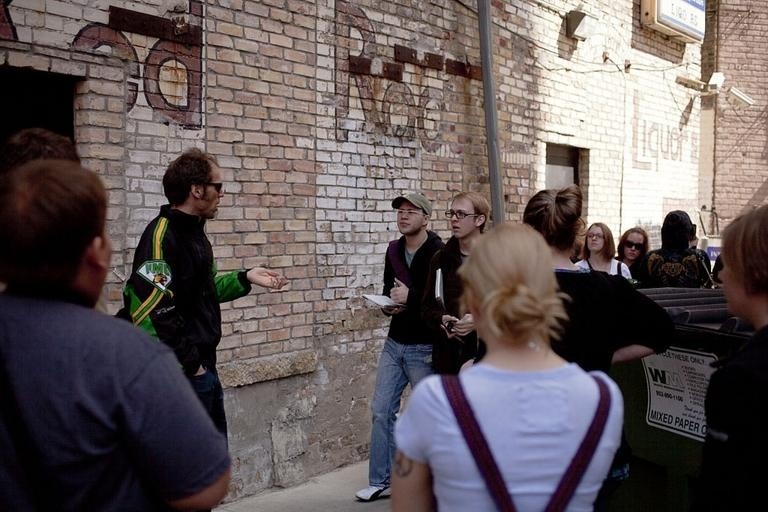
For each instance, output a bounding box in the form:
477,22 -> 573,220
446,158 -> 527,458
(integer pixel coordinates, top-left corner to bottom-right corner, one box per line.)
388,220 -> 624,512
523,186 -> 677,374
425,190 -> 491,372
578,211 -> 728,289
116,149 -> 289,447
0,160 -> 231,510
702,208 -> 766,509
0,127 -> 79,161
354,195 -> 445,501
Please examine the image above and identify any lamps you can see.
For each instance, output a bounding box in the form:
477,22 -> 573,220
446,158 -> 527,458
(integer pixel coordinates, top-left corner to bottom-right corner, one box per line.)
565,9 -> 600,42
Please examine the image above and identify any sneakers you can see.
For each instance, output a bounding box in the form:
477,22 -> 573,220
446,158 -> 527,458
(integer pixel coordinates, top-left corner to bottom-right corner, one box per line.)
355,484 -> 391,500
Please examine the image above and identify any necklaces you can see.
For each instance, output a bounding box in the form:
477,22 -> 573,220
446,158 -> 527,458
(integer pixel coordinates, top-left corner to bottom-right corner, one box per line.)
491,340 -> 564,359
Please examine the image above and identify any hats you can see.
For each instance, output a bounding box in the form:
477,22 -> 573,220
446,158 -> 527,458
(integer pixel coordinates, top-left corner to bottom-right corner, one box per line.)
663,211 -> 694,235
391,192 -> 432,217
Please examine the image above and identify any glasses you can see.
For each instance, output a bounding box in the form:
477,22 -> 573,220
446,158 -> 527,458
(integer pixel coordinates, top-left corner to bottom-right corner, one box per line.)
444,210 -> 479,220
589,232 -> 604,239
395,210 -> 425,218
203,182 -> 223,193
624,240 -> 643,250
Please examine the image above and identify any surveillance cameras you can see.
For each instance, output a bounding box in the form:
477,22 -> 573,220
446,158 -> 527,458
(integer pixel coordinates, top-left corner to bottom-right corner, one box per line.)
730,85 -> 754,108
708,70 -> 725,95
